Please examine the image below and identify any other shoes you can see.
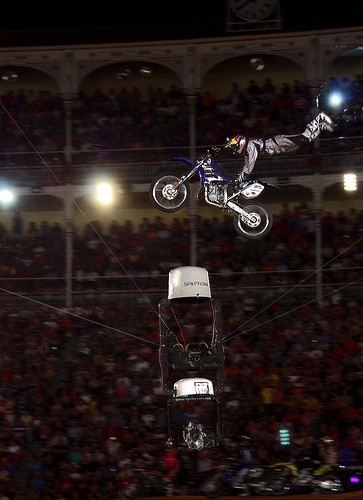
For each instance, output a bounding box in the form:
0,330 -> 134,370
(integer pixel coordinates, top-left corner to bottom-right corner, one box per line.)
317,112 -> 333,125
320,122 -> 334,133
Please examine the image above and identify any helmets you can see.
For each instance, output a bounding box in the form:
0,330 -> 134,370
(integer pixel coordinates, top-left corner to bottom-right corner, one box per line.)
225,135 -> 246,155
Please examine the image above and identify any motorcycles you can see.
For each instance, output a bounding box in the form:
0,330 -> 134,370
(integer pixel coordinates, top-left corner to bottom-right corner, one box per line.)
201,437 -> 348,496
149,146 -> 283,242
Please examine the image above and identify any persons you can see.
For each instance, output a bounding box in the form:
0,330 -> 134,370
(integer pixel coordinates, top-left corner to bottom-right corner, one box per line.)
319,73 -> 363,152
71,84 -> 191,163
0,290 -> 363,500
224,112 -> 334,182
0,88 -> 66,180
320,207 -> 363,287
73,216 -> 190,291
0,212 -> 67,296
195,77 -> 313,159
195,200 -> 318,297
